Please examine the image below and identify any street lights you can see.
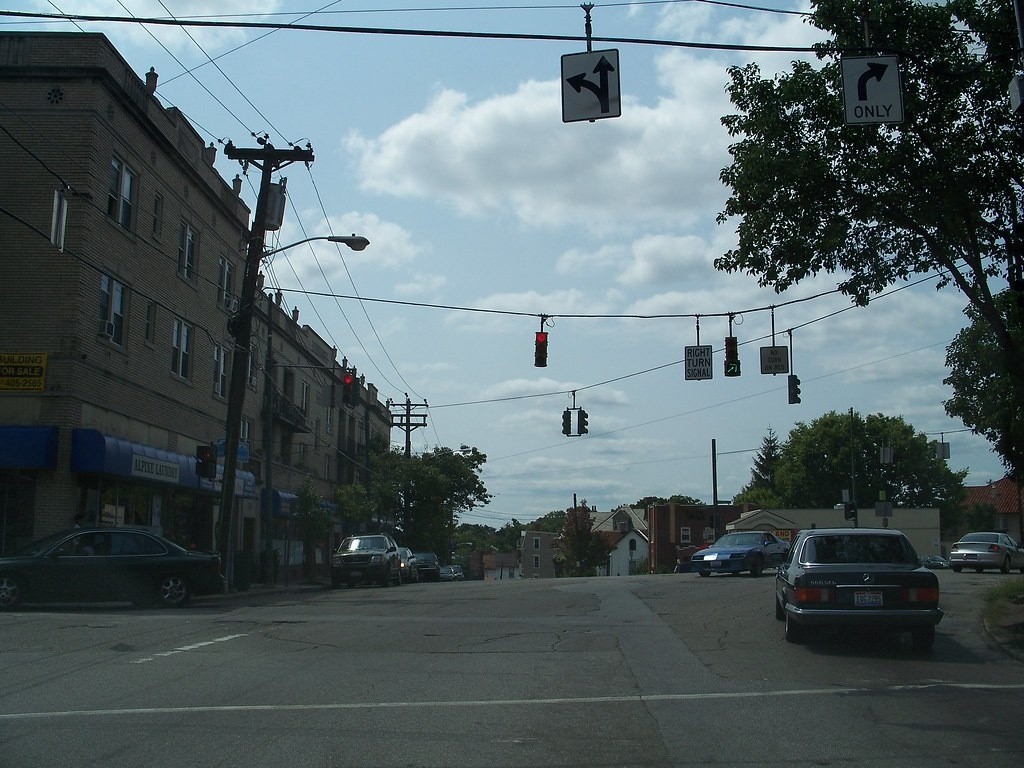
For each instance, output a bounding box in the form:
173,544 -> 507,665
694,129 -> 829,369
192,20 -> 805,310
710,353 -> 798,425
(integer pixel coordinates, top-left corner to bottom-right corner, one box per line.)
215,234 -> 370,589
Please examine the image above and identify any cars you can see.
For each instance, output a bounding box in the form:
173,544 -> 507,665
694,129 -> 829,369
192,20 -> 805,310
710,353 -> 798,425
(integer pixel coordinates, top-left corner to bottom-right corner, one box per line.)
398,547 -> 464,583
770,528 -> 944,650
922,555 -> 950,570
691,531 -> 793,578
949,532 -> 1024,574
0,528 -> 225,613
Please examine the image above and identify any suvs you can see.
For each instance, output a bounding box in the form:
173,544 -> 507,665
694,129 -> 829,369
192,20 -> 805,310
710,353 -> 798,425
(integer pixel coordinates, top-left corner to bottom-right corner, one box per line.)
331,533 -> 402,589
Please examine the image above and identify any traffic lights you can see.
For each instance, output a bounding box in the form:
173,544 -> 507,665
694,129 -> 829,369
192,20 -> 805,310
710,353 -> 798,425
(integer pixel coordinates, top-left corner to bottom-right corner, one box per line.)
724,337 -> 741,377
578,410 -> 588,434
196,446 -> 217,478
562,411 -> 571,434
534,332 -> 548,367
788,375 -> 801,404
342,375 -> 353,404
